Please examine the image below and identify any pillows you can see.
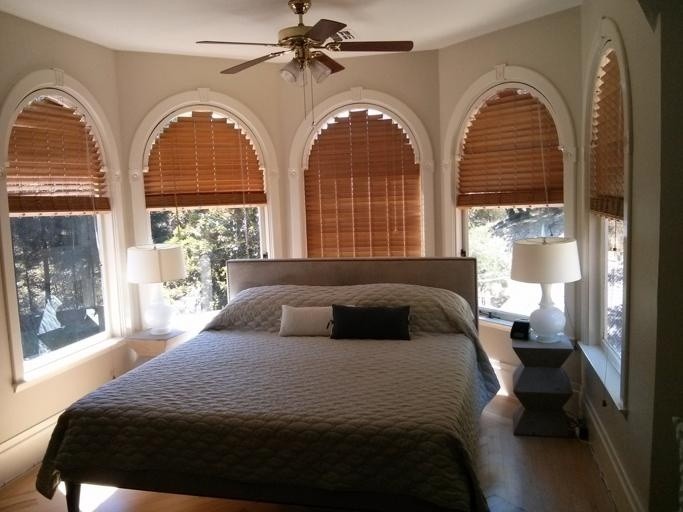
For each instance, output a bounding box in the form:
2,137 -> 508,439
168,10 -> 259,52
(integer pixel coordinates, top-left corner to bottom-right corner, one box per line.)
331,304 -> 411,341
278,305 -> 359,338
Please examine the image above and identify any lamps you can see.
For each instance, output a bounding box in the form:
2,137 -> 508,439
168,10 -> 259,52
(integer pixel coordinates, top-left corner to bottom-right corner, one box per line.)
126,243 -> 187,336
278,49 -> 332,85
510,237 -> 581,342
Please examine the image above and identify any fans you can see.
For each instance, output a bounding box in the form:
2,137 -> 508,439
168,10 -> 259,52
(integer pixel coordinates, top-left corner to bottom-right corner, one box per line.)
195,0 -> 413,76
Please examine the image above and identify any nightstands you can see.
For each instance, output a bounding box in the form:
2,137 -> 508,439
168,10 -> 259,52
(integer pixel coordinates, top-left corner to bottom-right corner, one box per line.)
511,339 -> 579,437
126,328 -> 185,367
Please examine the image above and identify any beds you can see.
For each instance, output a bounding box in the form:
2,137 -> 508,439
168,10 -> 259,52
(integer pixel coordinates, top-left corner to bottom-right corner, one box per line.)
37,257 -> 491,511
